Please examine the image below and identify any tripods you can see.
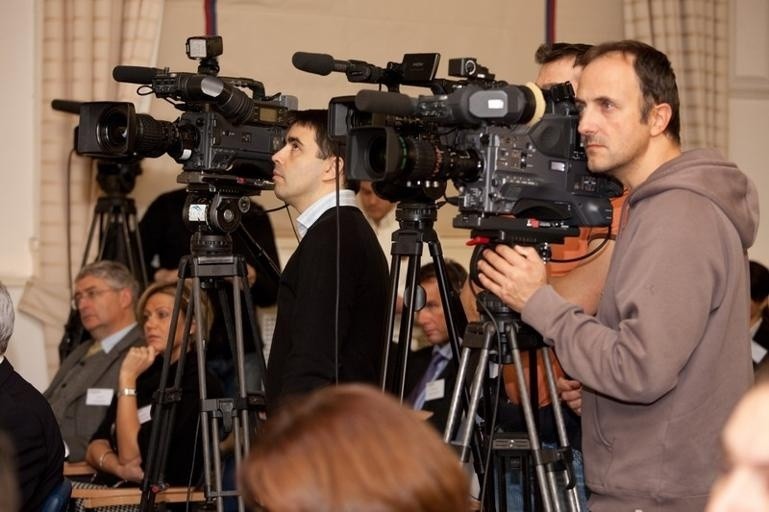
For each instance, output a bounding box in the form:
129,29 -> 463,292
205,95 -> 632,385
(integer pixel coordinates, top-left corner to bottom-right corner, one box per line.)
445,292 -> 583,512
139,173 -> 273,512
58,196 -> 150,368
380,202 -> 501,512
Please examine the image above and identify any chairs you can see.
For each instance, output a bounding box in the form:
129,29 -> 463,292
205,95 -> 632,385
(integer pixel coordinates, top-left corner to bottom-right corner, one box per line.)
47,461 -> 212,512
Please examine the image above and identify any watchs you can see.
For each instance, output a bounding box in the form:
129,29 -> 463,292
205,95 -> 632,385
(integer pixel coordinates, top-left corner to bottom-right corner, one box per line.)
117,387 -> 138,394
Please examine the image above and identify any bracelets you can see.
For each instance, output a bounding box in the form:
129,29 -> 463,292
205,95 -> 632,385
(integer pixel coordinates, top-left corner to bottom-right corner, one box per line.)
101,452 -> 112,467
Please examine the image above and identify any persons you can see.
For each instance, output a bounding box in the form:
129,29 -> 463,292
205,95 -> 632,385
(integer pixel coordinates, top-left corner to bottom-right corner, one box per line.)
263,109 -> 389,412
85,271 -> 215,494
743,254 -> 767,363
135,194 -> 279,381
234,384 -> 475,511
474,38 -> 761,512
40,262 -> 145,462
488,43 -> 636,458
392,259 -> 470,440
0,276 -> 65,512
360,162 -> 419,399
706,366 -> 769,512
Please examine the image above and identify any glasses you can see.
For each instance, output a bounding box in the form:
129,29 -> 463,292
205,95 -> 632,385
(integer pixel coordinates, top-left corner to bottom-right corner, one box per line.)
71,287 -> 121,311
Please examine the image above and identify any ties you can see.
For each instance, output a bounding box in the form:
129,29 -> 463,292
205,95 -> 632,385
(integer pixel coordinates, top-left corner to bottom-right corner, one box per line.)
408,352 -> 445,407
